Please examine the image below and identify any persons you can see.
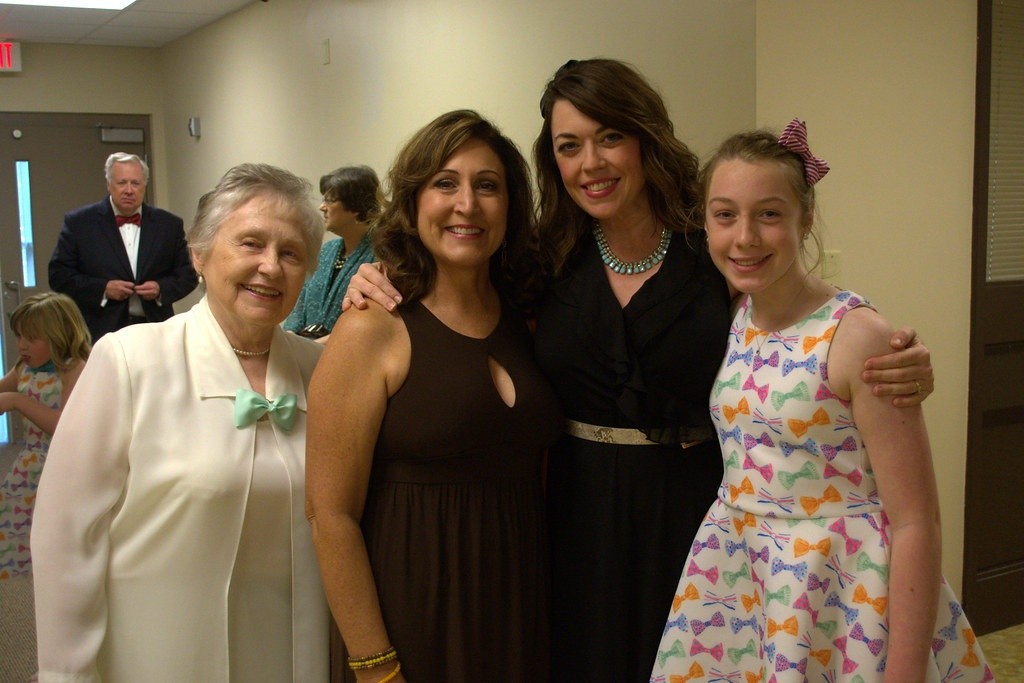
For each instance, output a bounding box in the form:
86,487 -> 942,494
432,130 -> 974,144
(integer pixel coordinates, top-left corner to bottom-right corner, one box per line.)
1,292 -> 95,578
28,162 -> 333,683
339,55 -> 934,682
648,128 -> 997,683
304,107 -> 560,683
47,151 -> 200,347
284,164 -> 384,342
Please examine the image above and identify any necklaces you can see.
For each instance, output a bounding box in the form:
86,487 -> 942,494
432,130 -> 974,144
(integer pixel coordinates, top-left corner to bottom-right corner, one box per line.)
231,346 -> 270,357
750,270 -> 810,355
589,214 -> 674,274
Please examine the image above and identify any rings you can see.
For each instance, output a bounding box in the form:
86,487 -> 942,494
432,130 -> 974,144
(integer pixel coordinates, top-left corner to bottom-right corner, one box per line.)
915,380 -> 922,394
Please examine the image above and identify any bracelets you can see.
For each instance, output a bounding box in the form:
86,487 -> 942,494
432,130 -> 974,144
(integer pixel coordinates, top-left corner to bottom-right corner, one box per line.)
345,647 -> 400,683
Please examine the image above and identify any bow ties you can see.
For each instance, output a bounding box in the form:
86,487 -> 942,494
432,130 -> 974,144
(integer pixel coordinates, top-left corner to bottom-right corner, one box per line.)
116,213 -> 142,227
234,389 -> 300,432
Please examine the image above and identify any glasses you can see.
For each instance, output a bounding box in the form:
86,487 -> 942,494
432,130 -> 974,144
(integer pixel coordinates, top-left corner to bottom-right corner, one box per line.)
322,198 -> 339,204
